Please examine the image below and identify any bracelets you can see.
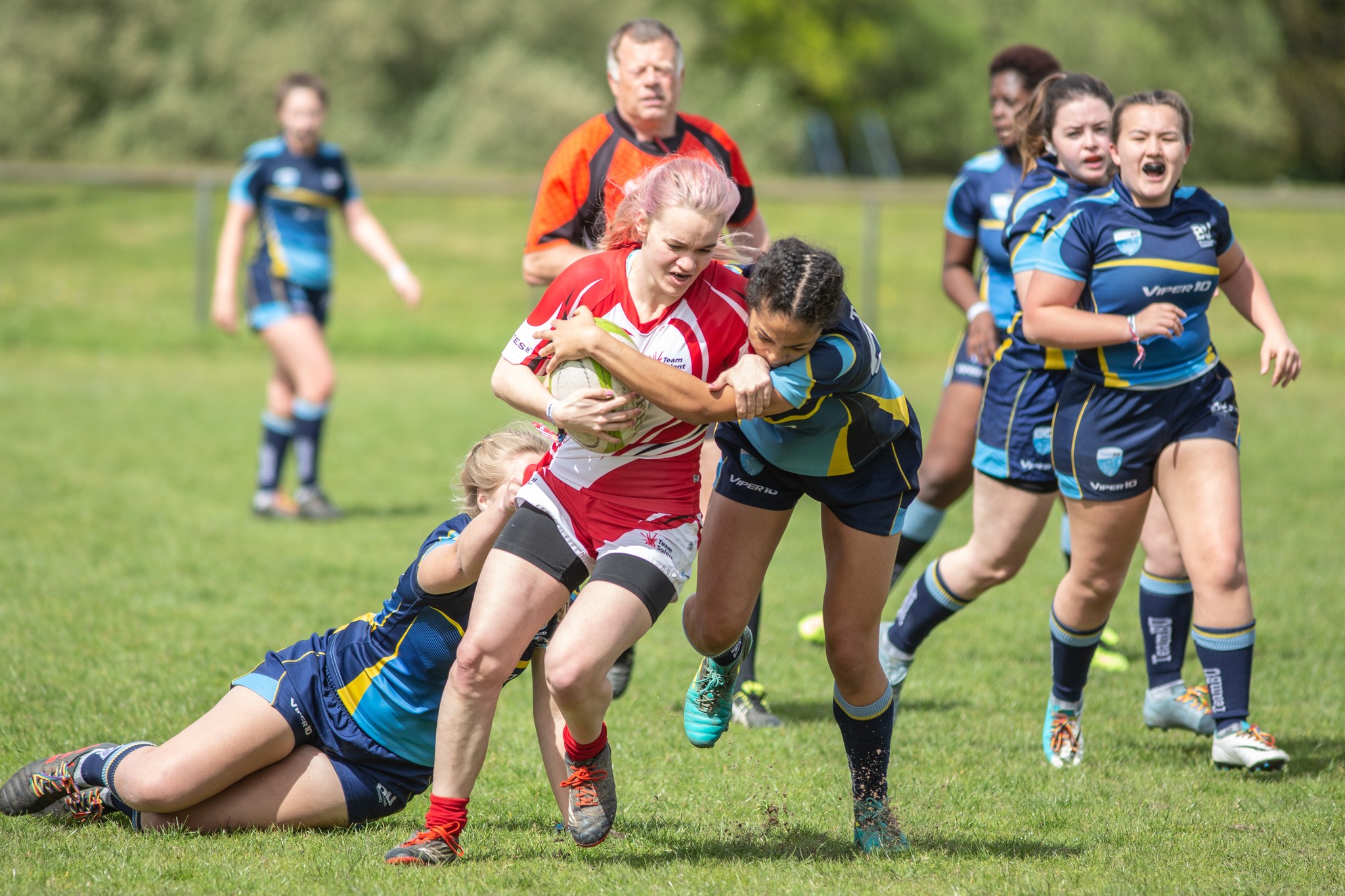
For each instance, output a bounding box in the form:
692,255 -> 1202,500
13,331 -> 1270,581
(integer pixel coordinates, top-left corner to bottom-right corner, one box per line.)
546,398 -> 556,424
965,303 -> 990,322
1126,314 -> 1145,373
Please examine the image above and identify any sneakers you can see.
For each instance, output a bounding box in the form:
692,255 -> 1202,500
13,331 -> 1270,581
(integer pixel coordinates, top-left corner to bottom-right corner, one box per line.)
684,624 -> 753,748
383,821 -> 464,867
0,743 -> 119,830
854,797 -> 914,858
1210,720 -> 1290,772
1041,689 -> 1084,770
564,738 -> 617,848
1144,680 -> 1216,737
731,680 -> 785,730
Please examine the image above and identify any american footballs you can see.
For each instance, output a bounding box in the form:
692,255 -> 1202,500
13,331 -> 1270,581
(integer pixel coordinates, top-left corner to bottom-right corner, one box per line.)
548,317 -> 649,454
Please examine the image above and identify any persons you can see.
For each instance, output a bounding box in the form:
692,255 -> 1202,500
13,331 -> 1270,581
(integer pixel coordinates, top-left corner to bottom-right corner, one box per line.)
210,71 -> 417,522
532,237 -> 913,857
524,20 -> 776,727
1024,91 -> 1301,772
799,44 -> 1129,670
879,73 -> 1221,733
0,430 -> 582,834
385,158 -> 770,871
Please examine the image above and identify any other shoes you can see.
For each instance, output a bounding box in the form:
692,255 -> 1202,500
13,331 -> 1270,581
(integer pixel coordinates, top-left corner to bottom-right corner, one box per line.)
251,482 -> 338,522
878,621 -> 915,727
798,611 -> 825,646
606,644 -> 634,698
1091,628 -> 1129,673
